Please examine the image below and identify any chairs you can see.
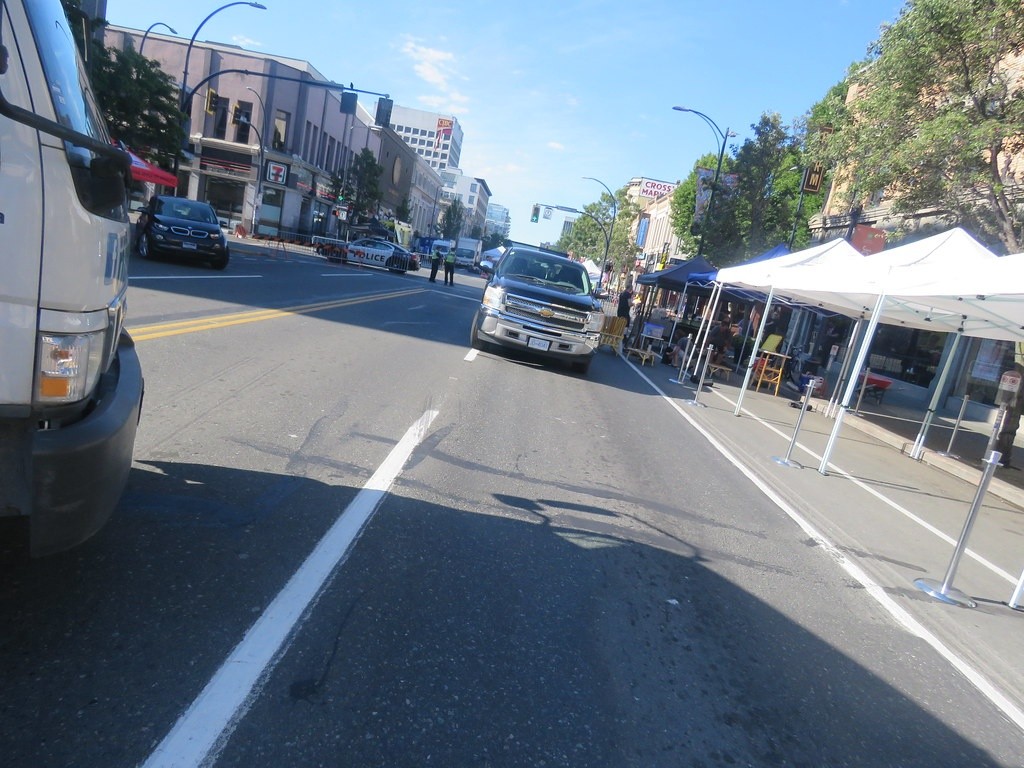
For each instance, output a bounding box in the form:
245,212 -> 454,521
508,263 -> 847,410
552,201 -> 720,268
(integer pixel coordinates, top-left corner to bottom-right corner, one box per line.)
598,314 -> 627,356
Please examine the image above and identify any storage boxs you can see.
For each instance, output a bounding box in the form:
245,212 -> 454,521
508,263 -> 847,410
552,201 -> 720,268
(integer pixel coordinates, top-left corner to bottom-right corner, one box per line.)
643,321 -> 668,338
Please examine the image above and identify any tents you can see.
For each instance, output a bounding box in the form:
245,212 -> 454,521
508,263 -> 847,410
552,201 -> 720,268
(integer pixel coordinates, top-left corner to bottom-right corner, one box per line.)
109,136 -> 177,211
689,237 -> 868,416
737,228 -> 998,471
820,251 -> 1024,610
482,246 -> 507,261
668,243 -> 845,384
628,254 -> 738,354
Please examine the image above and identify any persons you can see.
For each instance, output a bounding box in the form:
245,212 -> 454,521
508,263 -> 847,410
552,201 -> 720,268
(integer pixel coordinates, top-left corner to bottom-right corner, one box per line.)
706,317 -> 733,364
429,245 -> 443,283
737,305 -> 777,344
615,282 -> 635,340
666,329 -> 696,370
444,247 -> 455,286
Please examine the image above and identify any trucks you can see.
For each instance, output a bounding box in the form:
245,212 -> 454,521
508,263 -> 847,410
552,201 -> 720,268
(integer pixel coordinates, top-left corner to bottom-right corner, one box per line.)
454,237 -> 483,269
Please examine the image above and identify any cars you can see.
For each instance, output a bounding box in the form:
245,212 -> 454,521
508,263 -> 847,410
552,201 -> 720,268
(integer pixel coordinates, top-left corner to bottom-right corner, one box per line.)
134,195 -> 230,271
313,236 -> 421,275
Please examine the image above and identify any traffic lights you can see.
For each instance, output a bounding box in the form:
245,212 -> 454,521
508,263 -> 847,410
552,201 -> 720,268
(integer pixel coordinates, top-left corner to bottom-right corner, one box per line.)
529,204 -> 541,223
335,210 -> 339,216
338,188 -> 343,201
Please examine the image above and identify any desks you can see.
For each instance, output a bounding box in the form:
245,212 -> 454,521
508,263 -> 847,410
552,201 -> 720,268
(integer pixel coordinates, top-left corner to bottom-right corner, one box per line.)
625,347 -> 656,366
641,333 -> 665,354
748,348 -> 792,396
706,362 -> 732,381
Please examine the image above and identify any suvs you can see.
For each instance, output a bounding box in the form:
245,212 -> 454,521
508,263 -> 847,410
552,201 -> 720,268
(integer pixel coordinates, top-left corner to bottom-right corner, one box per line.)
469,246 -> 609,375
0,0 -> 149,567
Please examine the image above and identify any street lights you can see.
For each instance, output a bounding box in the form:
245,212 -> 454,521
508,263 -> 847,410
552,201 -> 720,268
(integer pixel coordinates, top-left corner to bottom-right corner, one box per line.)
169,2 -> 266,197
139,22 -> 179,59
582,175 -> 616,298
659,106 -> 737,365
231,86 -> 267,239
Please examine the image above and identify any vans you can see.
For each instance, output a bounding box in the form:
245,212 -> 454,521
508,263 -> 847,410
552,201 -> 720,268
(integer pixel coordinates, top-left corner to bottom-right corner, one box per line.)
430,239 -> 451,262
